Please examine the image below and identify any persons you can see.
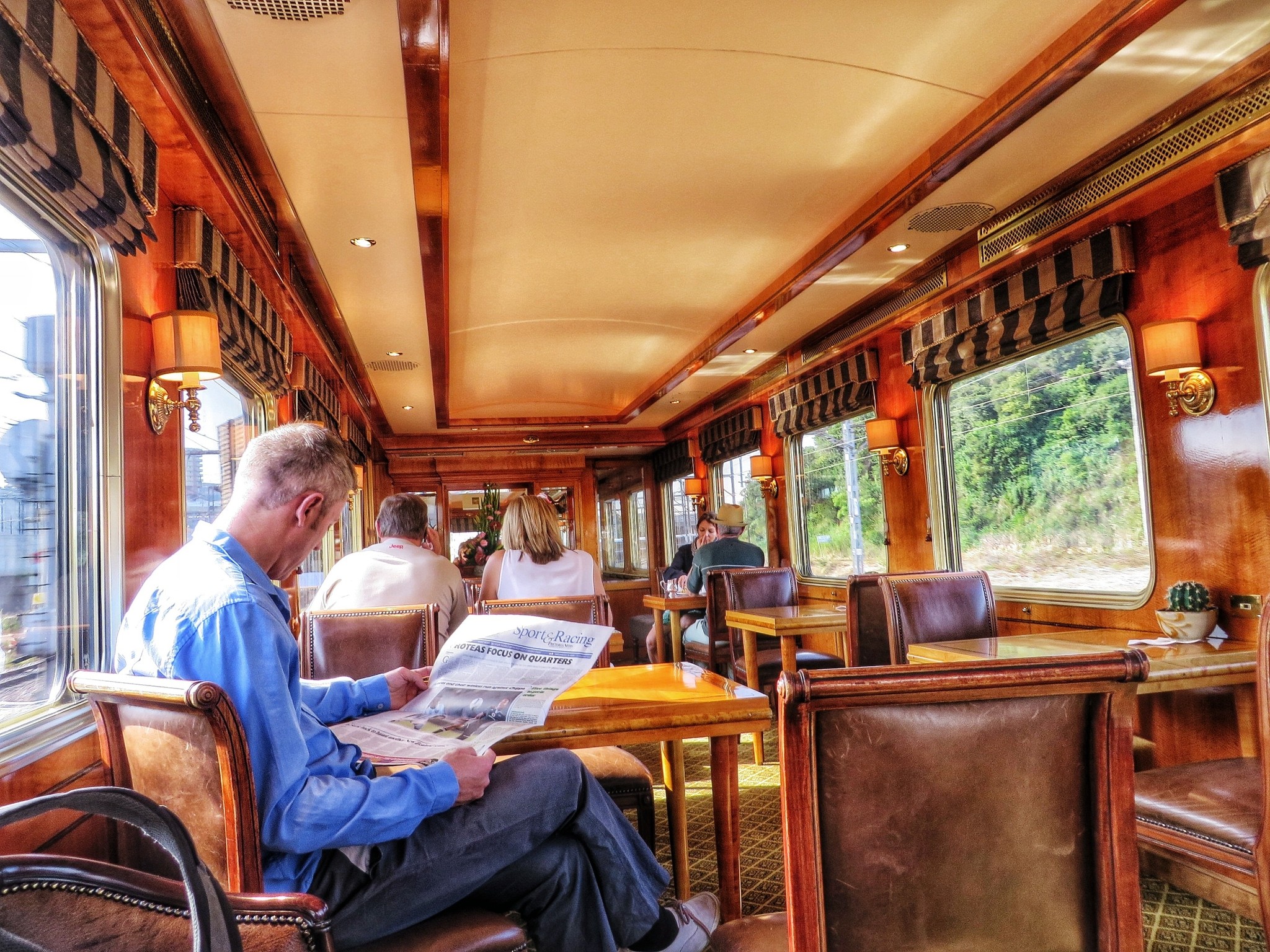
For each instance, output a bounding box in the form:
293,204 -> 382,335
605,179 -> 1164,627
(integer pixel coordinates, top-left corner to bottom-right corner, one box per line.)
645,512 -> 719,664
297,492 -> 469,655
681,503 -> 765,681
113,420 -> 721,952
476,496 -> 613,629
391,689 -> 510,741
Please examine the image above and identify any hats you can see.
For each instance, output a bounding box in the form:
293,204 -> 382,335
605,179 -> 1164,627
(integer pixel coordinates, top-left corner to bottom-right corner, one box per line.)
709,502 -> 748,527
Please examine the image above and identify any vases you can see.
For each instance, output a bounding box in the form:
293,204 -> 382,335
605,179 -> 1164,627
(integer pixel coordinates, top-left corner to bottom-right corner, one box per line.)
473,564 -> 485,577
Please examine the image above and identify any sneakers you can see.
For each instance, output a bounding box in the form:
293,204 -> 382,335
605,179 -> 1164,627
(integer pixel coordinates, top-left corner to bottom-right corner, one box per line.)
653,889 -> 720,951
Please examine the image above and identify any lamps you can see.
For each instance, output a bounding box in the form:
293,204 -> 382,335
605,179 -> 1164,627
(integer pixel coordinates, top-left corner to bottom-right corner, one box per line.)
864,418 -> 909,476
684,478 -> 705,512
348,465 -> 363,512
146,310 -> 223,436
1140,317 -> 1215,418
750,455 -> 778,498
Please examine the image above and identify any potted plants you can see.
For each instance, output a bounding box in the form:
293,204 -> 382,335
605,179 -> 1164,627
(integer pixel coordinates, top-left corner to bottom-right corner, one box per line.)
1154,578 -> 1219,641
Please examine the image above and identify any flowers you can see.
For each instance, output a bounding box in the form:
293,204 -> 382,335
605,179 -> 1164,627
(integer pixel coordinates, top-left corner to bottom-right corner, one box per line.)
459,480 -> 503,567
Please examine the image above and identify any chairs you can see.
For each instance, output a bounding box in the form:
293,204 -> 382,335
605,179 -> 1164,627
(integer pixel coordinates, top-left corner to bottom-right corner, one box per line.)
847,570 -> 1157,769
65,667 -> 536,952
0,852 -> 334,952
480,593 -> 656,858
299,604 -> 439,680
707,651 -> 1153,952
627,567 -> 847,766
1132,594 -> 1270,952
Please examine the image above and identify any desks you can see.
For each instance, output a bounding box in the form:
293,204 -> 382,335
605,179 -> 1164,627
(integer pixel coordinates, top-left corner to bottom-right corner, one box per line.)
490,659 -> 774,923
907,628 -> 1258,759
643,591 -> 707,662
725,603 -> 847,765
